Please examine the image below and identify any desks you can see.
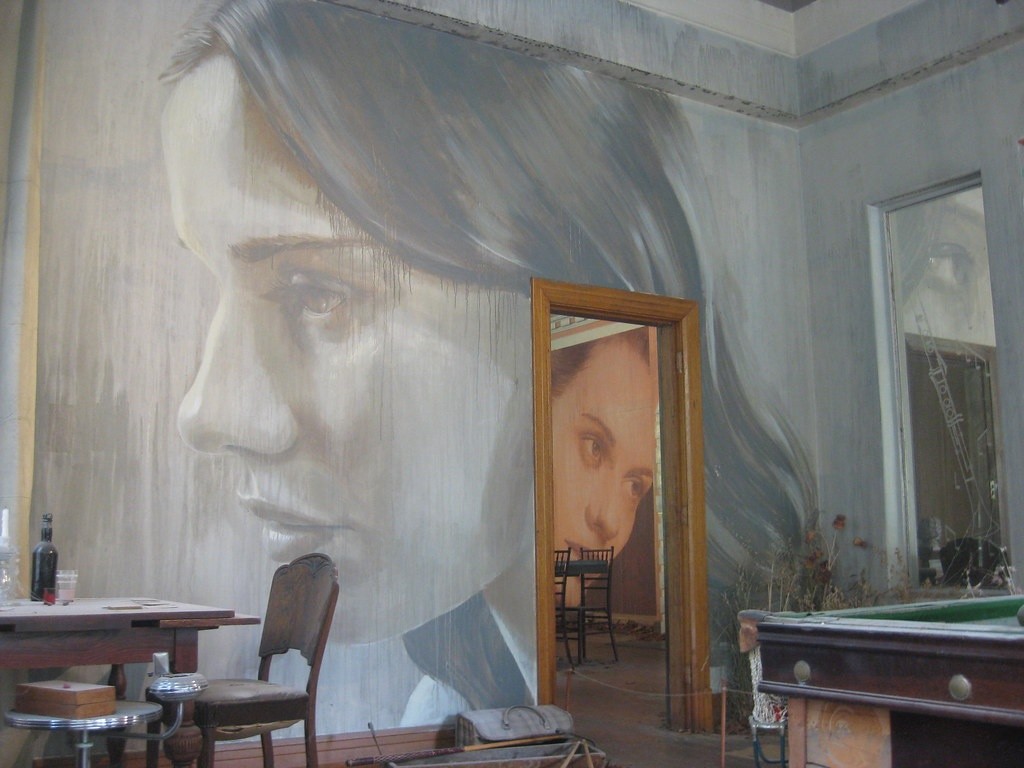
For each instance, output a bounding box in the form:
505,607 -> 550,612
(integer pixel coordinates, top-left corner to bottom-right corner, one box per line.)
554,560 -> 609,576
737,595 -> 1024,768
0,597 -> 262,768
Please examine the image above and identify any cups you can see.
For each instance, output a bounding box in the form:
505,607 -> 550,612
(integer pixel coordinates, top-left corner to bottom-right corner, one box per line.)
43,588 -> 57,605
57,567 -> 79,605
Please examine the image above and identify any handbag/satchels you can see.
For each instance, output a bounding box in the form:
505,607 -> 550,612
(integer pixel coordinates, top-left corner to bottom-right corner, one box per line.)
456,704 -> 575,749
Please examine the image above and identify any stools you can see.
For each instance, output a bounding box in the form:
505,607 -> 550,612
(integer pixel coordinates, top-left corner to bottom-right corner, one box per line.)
4,673 -> 210,768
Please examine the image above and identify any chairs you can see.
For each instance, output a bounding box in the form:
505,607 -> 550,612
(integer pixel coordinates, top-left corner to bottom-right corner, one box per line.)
144,553 -> 340,768
555,546 -> 619,671
939,537 -> 1016,594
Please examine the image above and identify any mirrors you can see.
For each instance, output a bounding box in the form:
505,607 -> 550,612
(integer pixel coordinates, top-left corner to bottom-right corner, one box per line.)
883,175 -> 1011,589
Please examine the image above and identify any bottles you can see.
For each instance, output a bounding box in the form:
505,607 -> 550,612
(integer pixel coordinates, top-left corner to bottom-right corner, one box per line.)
30,513 -> 58,601
1,536 -> 22,614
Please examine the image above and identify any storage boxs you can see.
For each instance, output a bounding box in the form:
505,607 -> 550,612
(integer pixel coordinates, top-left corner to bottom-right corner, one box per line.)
14,680 -> 117,720
